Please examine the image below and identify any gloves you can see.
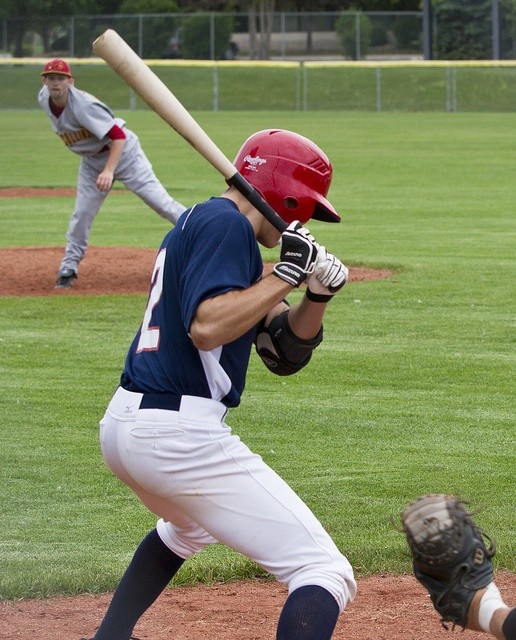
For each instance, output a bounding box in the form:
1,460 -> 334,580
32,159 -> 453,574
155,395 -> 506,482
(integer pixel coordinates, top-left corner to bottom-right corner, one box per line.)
272,220 -> 317,287
308,248 -> 348,302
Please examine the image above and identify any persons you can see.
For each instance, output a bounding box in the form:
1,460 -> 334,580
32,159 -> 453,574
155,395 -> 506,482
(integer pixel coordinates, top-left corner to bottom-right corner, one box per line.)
402,495 -> 516,640
87,129 -> 359,640
35,59 -> 189,291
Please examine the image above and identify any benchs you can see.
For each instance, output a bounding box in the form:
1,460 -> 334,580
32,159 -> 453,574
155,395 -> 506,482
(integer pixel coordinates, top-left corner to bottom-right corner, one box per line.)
229,32 -> 425,61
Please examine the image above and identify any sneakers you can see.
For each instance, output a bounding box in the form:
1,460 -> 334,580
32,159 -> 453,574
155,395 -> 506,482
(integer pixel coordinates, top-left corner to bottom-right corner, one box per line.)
54,266 -> 78,288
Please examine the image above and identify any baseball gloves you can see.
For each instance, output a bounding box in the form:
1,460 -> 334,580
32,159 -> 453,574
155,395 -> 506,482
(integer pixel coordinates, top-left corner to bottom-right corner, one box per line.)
401,493 -> 496,632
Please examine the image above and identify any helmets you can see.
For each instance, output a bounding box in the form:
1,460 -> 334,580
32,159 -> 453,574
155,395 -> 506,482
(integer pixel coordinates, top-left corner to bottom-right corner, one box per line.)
232,129 -> 340,230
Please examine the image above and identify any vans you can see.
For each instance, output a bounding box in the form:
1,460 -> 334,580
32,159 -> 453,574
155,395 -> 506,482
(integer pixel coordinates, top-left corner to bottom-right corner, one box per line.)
11,30 -> 45,57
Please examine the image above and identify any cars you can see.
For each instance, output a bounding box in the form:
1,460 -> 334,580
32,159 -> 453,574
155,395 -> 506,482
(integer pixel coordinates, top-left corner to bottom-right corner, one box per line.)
159,25 -> 239,60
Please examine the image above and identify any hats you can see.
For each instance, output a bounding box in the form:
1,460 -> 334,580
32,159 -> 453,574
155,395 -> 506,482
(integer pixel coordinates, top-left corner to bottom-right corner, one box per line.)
41,61 -> 71,78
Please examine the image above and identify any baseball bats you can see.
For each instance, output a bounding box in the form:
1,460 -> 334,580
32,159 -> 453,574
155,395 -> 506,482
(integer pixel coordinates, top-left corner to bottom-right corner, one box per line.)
92,28 -> 288,235
